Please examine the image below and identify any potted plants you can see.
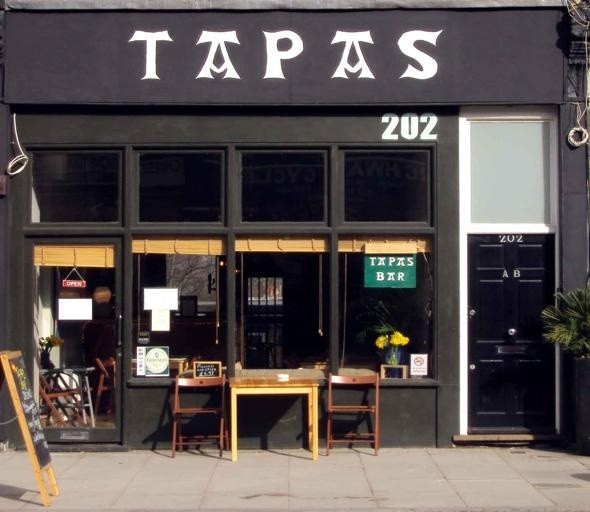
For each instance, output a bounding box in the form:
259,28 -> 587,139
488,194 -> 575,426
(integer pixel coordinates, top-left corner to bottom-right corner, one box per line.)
540,287 -> 590,456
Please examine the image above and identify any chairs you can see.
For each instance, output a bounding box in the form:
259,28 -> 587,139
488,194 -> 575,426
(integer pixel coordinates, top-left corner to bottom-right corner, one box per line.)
171,374 -> 229,457
38,356 -> 118,428
326,372 -> 380,456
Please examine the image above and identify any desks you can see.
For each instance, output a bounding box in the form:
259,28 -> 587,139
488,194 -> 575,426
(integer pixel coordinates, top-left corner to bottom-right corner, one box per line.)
228,376 -> 320,463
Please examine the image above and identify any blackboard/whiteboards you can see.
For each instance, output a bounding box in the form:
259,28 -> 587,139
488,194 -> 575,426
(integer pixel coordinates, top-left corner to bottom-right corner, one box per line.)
1,350 -> 52,472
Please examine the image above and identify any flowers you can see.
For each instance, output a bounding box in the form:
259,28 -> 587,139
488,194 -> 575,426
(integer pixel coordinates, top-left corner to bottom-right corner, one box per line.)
38,334 -> 65,354
365,299 -> 410,361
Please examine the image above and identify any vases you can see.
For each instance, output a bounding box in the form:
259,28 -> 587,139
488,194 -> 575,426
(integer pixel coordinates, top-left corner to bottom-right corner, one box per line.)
40,351 -> 56,369
384,344 -> 402,365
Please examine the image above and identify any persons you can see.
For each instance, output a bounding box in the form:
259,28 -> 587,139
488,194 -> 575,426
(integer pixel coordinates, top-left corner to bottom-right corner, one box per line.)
85,303 -> 116,414
